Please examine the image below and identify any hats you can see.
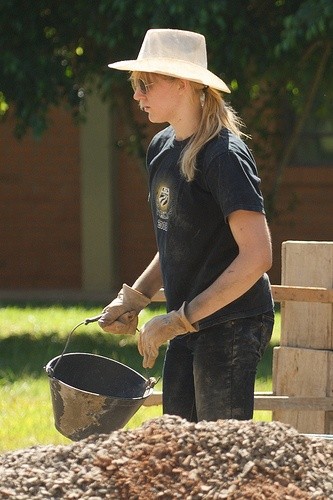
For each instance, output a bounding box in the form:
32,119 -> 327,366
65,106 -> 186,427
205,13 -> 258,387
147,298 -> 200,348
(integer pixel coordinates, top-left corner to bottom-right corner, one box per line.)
108,29 -> 231,93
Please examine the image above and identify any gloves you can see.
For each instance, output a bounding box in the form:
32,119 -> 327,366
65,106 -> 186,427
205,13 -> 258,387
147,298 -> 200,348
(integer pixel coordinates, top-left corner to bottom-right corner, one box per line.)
98,284 -> 152,335
138,301 -> 199,368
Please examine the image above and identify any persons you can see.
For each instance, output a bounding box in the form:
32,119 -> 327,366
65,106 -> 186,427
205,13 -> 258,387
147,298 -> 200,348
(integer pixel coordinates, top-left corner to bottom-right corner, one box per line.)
97,29 -> 276,424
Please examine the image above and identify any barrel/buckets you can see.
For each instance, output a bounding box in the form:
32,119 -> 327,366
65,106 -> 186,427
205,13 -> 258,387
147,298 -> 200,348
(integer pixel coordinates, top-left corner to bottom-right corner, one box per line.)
45,315 -> 162,442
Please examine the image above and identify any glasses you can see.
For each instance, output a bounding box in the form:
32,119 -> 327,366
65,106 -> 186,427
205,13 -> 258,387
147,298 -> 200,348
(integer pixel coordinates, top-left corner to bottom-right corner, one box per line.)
131,78 -> 166,95
